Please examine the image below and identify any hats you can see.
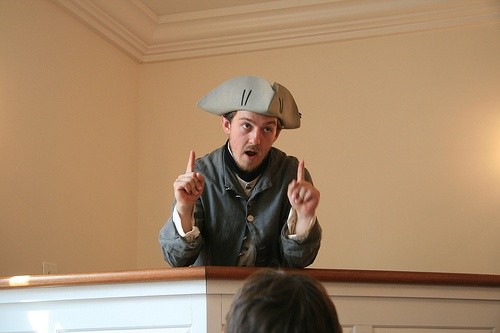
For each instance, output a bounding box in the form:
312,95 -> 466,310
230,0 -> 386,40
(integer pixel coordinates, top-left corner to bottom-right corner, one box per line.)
198,76 -> 300,129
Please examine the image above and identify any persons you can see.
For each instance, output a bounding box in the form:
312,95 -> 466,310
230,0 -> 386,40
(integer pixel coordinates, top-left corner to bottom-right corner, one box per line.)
225,266 -> 342,333
159,73 -> 323,269
